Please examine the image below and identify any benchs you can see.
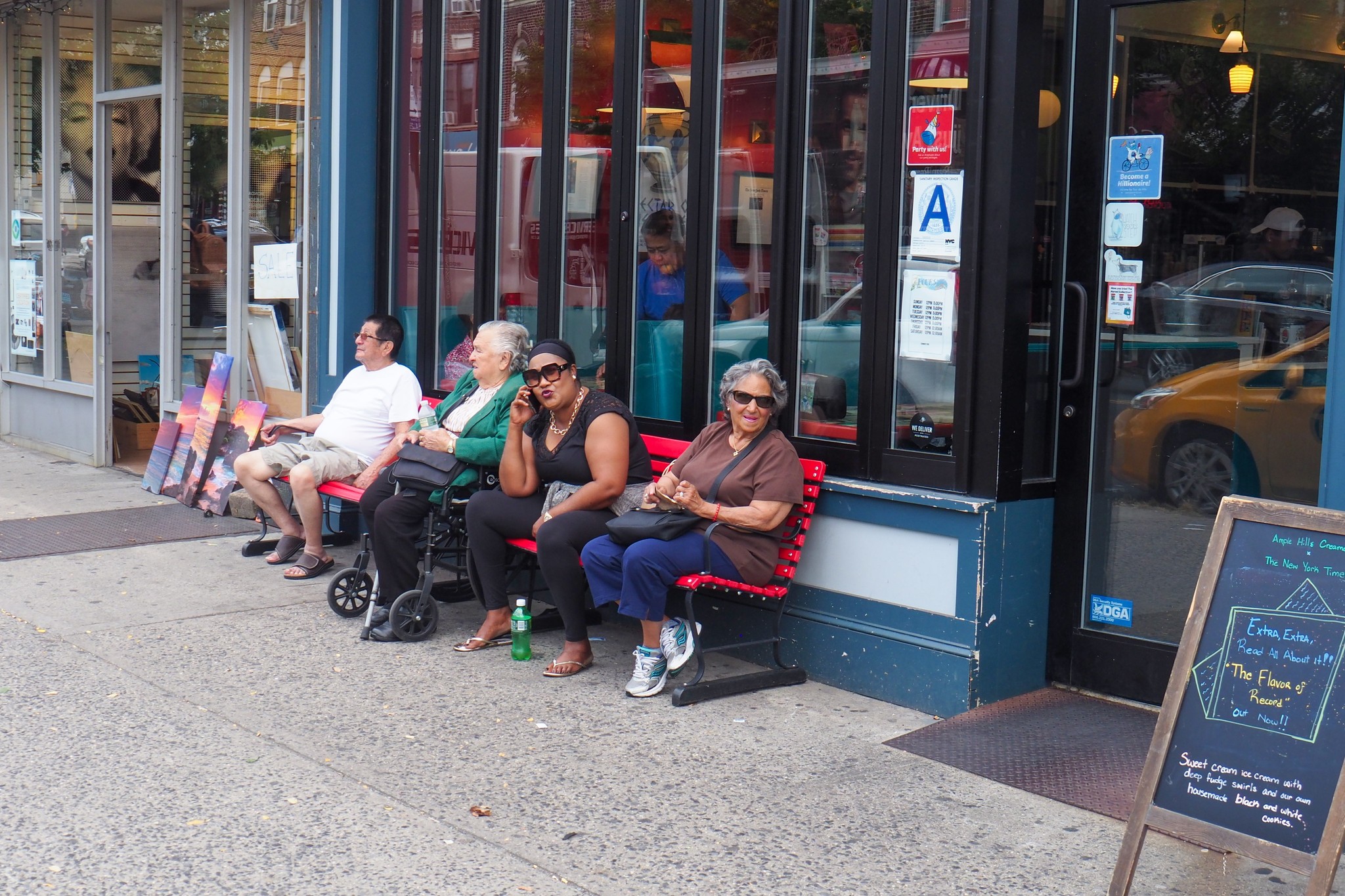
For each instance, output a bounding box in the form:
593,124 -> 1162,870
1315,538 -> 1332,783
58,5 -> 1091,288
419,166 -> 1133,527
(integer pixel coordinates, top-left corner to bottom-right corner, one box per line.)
466,434 -> 828,706
400,305 -> 860,421
242,396 -> 473,602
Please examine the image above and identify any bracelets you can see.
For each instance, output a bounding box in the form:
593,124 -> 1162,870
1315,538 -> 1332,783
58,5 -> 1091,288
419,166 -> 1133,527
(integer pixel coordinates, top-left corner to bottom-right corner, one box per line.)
712,503 -> 721,522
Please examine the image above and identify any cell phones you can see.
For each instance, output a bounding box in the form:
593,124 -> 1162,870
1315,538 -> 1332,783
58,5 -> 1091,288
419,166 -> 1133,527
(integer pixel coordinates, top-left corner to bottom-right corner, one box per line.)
523,387 -> 541,414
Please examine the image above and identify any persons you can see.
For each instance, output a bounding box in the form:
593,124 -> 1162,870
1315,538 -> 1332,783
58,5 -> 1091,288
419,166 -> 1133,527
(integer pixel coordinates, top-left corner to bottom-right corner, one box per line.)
1241,206 -> 1330,349
440,286 -> 508,381
79,231 -> 95,272
595,208 -> 750,378
360,320 -> 532,641
580,358 -> 804,699
647,202 -> 674,235
233,315 -> 423,579
32,59 -> 164,203
451,339 -> 655,677
810,78 -> 869,226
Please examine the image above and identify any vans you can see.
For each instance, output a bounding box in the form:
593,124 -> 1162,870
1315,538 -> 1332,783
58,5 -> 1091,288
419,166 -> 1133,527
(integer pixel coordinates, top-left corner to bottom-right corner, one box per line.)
400,145 -> 760,370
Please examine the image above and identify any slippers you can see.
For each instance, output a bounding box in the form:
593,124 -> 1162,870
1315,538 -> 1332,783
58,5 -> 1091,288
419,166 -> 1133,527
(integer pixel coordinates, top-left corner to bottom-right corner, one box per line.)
266,534 -> 306,565
453,637 -> 513,652
542,658 -> 593,677
283,552 -> 335,579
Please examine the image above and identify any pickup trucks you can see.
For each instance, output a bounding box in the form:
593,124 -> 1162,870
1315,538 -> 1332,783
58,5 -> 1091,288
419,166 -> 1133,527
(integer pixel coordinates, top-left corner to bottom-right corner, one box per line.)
706,275 -> 1261,417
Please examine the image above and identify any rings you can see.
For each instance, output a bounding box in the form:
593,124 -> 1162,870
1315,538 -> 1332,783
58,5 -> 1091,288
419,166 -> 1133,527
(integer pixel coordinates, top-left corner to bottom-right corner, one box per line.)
680,491 -> 683,498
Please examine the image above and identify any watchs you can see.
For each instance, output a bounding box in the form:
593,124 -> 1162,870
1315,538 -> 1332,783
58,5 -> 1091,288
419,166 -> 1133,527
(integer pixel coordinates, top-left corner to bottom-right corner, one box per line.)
447,439 -> 454,455
544,511 -> 553,524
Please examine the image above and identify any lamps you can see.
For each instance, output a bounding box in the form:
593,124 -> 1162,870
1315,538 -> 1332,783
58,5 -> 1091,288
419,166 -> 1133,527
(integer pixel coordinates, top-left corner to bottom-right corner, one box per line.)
1229,0 -> 1254,93
1212,13 -> 1248,53
267,29 -> 284,50
191,10 -> 213,45
596,34 -> 686,114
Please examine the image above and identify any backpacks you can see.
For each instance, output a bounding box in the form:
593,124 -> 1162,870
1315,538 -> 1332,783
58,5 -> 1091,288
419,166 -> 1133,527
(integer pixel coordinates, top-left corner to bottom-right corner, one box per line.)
181,219 -> 228,289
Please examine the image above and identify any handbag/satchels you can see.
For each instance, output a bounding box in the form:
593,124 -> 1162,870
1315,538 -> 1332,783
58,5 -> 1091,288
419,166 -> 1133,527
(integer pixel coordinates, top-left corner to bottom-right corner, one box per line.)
605,506 -> 703,547
391,441 -> 468,493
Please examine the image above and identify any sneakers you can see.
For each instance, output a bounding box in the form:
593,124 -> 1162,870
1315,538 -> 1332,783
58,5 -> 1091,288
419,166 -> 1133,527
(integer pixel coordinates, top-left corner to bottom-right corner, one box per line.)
624,644 -> 669,697
659,616 -> 702,679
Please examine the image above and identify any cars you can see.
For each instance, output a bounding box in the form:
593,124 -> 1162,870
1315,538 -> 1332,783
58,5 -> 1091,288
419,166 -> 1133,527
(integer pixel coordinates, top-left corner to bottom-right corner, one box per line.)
1137,262 -> 1334,352
1113,327 -> 1329,515
192,216 -> 287,314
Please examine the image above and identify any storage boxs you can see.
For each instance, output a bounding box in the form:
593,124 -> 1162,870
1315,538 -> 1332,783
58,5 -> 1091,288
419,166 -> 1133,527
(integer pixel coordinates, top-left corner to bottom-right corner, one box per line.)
112,417 -> 159,450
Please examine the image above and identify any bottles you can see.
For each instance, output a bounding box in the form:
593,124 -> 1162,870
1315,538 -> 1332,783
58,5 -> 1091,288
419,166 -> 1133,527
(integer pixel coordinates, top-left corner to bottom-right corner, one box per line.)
418,400 -> 439,431
511,599 -> 532,660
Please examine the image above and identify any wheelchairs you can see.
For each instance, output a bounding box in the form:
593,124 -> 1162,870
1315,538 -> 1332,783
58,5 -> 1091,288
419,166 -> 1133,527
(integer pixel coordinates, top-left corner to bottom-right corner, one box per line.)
326,463 -> 588,642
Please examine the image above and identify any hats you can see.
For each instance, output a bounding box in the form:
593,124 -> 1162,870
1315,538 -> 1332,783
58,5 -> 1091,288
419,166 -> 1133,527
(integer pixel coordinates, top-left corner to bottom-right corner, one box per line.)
1250,206 -> 1306,234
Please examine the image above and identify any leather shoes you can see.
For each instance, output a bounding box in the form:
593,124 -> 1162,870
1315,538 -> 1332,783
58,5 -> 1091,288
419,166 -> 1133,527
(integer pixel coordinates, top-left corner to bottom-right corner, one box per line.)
369,610 -> 437,642
366,601 -> 402,628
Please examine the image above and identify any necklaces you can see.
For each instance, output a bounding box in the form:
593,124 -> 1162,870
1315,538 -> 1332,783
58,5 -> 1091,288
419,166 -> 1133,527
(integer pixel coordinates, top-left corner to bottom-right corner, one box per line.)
549,386 -> 584,436
731,433 -> 753,457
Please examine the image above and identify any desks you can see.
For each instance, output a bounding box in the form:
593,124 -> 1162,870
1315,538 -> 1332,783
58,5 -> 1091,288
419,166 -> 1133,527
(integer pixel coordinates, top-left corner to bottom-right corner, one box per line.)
717,402 -> 953,454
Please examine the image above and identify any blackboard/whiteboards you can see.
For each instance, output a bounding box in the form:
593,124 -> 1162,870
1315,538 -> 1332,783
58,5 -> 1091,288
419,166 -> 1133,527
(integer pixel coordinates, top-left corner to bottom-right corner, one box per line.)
1134,496 -> 1345,877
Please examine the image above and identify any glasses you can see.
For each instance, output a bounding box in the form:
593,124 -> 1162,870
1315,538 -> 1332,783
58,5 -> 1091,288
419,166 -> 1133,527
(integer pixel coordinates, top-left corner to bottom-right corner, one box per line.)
728,389 -> 776,408
522,362 -> 572,388
353,332 -> 388,341
